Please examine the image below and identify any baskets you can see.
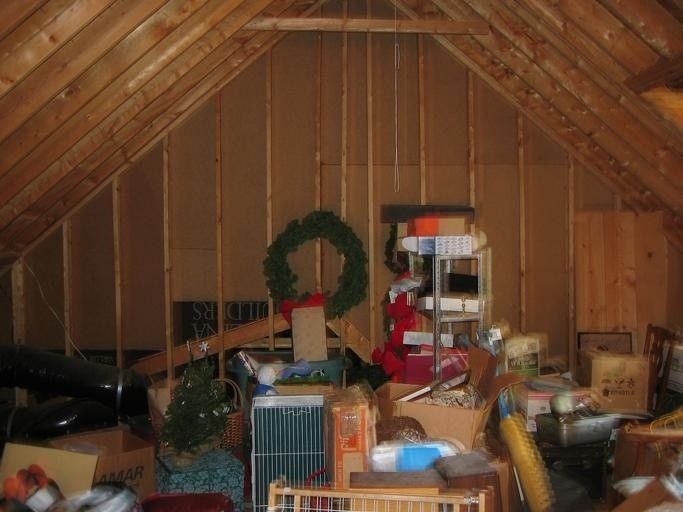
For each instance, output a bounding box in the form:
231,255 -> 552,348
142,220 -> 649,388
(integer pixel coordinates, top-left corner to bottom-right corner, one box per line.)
210,377 -> 245,450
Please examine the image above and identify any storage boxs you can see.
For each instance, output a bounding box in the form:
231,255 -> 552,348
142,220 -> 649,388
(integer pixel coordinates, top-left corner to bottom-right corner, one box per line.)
0,212 -> 683,512
